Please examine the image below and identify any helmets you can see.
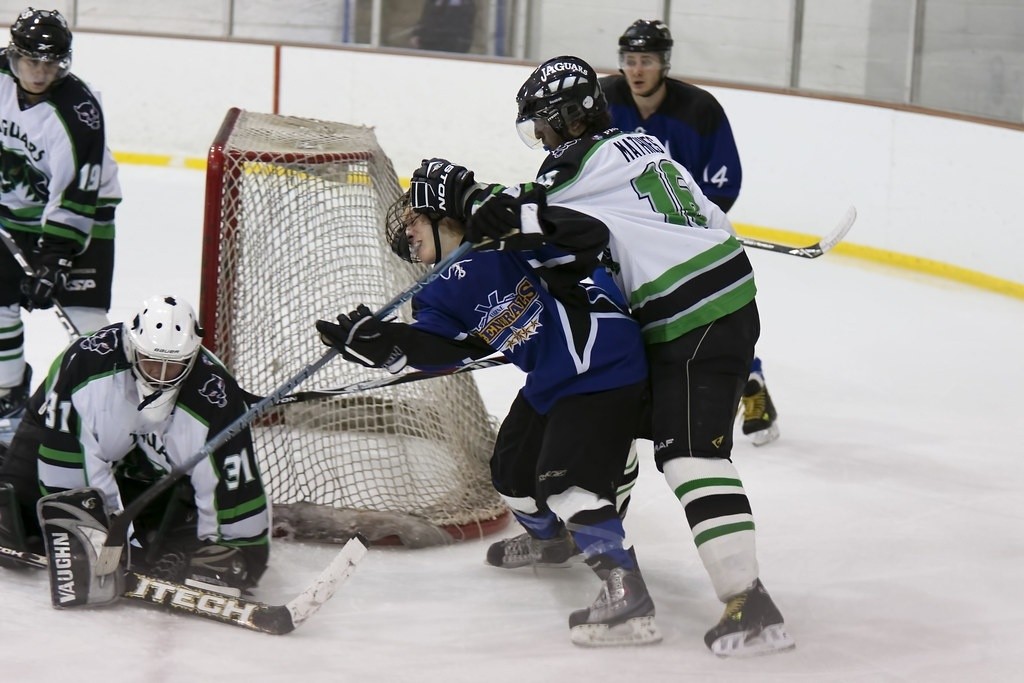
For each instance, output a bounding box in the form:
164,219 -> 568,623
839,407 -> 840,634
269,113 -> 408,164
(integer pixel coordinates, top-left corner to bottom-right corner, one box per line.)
618,21 -> 673,64
515,54 -> 606,135
128,298 -> 204,391
10,8 -> 72,59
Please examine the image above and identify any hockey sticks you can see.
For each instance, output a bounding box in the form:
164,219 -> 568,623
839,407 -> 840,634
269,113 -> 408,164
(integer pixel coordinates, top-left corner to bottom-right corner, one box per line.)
734,208 -> 858,261
0,533 -> 370,635
94,242 -> 473,577
0,225 -> 83,339
239,355 -> 513,410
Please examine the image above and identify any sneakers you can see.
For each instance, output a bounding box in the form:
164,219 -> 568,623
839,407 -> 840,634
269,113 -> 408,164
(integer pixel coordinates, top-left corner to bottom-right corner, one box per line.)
485,532 -> 577,577
704,577 -> 796,657
737,380 -> 779,446
568,545 -> 663,647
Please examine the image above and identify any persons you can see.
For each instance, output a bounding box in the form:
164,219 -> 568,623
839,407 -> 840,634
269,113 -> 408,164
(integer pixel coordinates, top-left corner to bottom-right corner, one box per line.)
0,297 -> 272,591
0,8 -> 123,414
594,19 -> 782,446
417,54 -> 795,658
317,181 -> 661,647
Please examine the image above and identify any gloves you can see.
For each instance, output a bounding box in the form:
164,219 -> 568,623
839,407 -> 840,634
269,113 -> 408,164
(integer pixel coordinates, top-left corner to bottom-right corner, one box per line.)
466,183 -> 522,243
412,158 -> 474,223
315,304 -> 414,373
21,234 -> 74,309
190,545 -> 269,590
